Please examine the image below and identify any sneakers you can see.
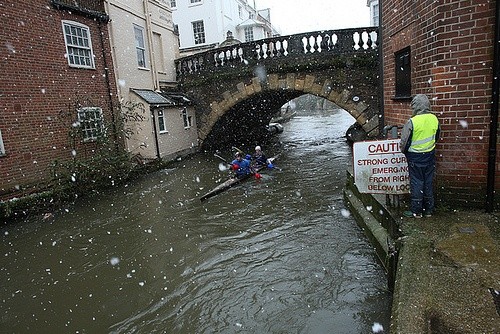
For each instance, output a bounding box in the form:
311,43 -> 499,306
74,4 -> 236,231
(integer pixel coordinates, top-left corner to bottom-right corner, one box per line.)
422,211 -> 431,217
403,210 -> 422,218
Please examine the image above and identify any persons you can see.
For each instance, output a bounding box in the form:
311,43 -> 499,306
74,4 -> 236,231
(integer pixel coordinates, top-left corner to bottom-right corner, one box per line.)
229,152 -> 260,180
245,145 -> 274,168
400,93 -> 441,218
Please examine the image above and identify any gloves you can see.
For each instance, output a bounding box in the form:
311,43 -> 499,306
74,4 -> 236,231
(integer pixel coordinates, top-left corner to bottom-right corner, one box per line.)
232,164 -> 239,170
246,154 -> 251,159
267,163 -> 273,168
255,173 -> 261,179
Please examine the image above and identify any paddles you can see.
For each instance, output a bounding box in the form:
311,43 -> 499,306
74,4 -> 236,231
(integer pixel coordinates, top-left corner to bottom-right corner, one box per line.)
232,145 -> 284,174
212,152 -> 262,178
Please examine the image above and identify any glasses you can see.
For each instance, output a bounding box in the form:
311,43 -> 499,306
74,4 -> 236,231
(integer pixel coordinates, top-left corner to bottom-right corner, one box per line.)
235,155 -> 239,158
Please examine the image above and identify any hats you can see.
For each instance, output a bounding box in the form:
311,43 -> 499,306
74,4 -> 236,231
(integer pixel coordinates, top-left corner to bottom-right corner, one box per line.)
234,152 -> 241,156
255,146 -> 261,151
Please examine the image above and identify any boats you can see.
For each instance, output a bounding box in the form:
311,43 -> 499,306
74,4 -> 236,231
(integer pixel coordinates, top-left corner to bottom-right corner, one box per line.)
199,152 -> 282,202
